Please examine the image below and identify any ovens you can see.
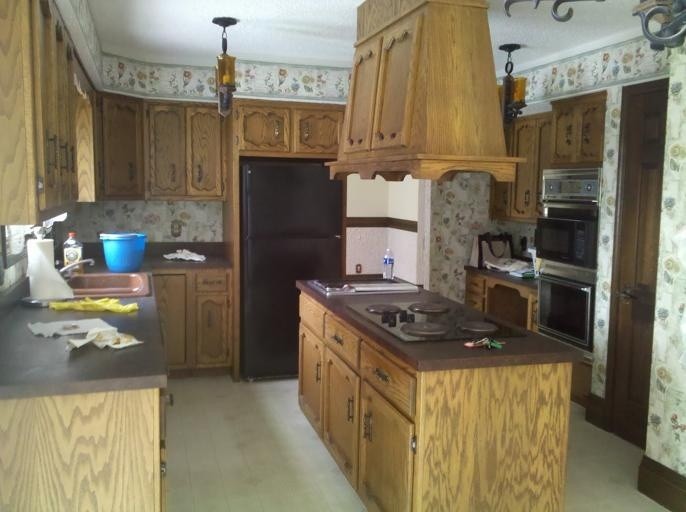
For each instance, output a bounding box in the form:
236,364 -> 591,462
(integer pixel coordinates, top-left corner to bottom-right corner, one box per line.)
537,276 -> 592,348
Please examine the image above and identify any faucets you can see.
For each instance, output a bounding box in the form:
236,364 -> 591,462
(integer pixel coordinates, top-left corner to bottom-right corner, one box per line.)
60,258 -> 95,274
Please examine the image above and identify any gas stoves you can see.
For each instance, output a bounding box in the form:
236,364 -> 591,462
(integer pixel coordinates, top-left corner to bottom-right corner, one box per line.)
347,300 -> 530,343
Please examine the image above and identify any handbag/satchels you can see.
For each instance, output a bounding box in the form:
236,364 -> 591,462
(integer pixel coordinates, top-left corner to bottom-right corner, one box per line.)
469,232 -> 514,269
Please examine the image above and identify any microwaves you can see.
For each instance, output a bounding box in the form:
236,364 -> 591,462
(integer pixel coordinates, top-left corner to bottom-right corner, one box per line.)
537,216 -> 599,269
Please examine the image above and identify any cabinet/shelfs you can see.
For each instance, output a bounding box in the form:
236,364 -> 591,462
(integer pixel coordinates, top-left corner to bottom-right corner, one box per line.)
550,90 -> 606,165
296,294 -> 325,435
145,98 -> 226,203
324,313 -> 413,512
234,98 -> 344,160
105,93 -> 144,200
0,0 -> 79,225
154,274 -> 232,372
489,114 -> 552,223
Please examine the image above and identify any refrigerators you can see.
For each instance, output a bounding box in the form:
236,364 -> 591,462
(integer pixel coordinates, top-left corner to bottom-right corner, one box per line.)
238,155 -> 343,381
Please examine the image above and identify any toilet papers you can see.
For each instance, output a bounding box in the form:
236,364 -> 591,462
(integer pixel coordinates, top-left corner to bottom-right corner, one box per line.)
26,239 -> 74,300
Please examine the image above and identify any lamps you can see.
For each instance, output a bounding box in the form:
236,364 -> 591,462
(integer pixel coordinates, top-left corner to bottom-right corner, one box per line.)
211,16 -> 237,119
495,43 -> 525,129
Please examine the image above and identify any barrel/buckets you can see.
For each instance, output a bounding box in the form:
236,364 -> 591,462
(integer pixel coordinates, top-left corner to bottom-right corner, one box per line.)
98,230 -> 146,273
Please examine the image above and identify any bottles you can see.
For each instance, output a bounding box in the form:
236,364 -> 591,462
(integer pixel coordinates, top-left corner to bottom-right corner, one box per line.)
383,249 -> 396,280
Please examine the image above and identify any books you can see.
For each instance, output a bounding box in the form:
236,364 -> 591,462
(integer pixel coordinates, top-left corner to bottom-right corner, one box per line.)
509,268 -> 534,279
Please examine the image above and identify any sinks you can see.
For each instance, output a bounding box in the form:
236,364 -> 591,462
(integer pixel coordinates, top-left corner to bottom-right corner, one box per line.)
66,271 -> 151,297
